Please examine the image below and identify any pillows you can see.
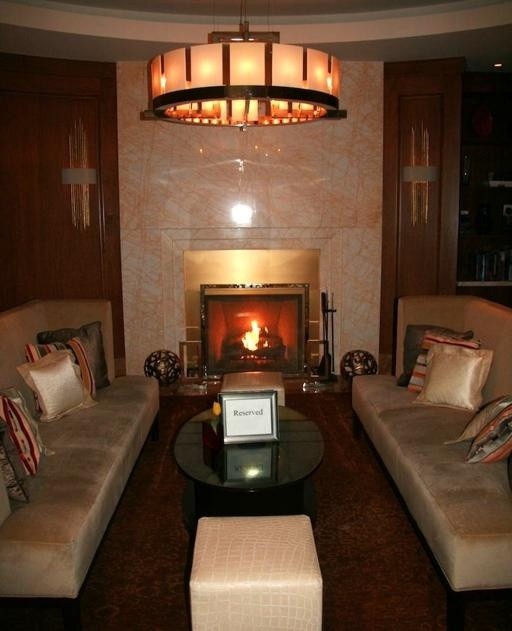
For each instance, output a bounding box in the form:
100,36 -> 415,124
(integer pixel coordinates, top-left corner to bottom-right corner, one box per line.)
0,319 -> 117,523
394,322 -> 512,465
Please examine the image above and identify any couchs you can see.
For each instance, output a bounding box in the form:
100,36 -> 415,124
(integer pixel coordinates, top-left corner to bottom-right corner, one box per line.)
0,299 -> 163,625
349,295 -> 512,631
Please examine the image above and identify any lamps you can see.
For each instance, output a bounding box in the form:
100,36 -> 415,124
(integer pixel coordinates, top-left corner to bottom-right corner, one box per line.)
140,3 -> 349,134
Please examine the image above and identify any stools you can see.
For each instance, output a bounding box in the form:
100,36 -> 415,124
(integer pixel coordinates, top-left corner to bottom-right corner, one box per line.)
188,514 -> 324,631
220,371 -> 287,408
